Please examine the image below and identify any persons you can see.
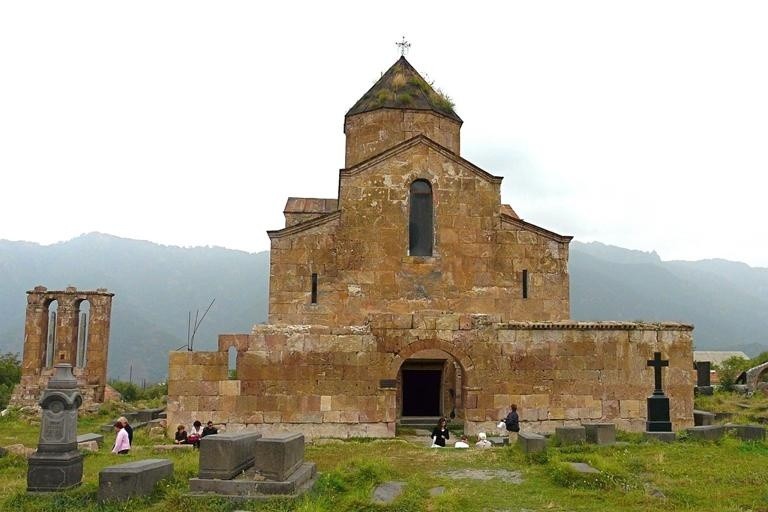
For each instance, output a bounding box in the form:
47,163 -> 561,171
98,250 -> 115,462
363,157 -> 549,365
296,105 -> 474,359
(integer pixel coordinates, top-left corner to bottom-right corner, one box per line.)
110,421 -> 131,454
186,420 -> 205,449
111,416 -> 134,453
454,435 -> 470,448
496,418 -> 508,436
503,403 -> 520,445
430,417 -> 450,448
173,423 -> 187,444
198,420 -> 218,447
476,432 -> 492,448
